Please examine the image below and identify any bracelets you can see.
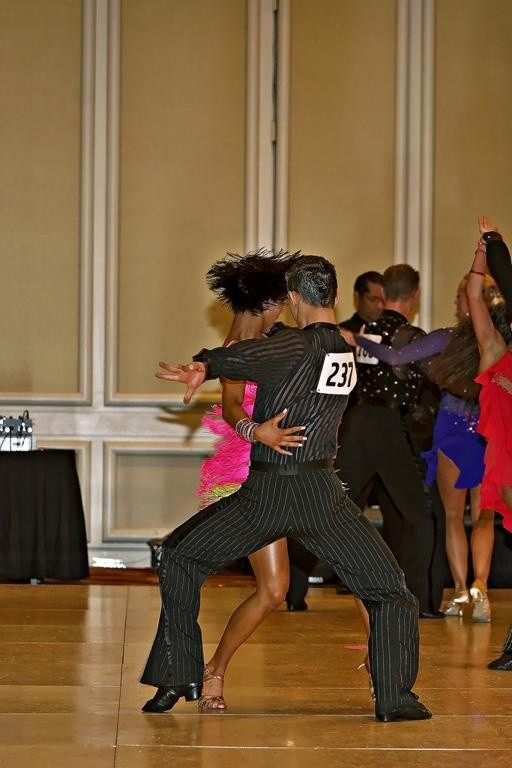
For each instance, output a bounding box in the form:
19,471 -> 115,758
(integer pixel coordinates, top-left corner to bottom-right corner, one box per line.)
232,419 -> 259,442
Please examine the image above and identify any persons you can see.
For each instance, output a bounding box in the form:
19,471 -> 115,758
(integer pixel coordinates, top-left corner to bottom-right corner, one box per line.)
138,256 -> 433,720
194,248 -> 418,711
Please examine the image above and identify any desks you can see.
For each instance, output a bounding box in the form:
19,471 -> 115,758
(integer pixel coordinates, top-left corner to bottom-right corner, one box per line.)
1,449 -> 80,585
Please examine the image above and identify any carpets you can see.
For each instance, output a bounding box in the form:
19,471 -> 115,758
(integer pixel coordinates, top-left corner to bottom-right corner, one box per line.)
2,565 -> 264,589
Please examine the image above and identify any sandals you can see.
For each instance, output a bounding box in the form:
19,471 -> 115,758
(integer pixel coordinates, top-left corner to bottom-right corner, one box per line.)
200,664 -> 227,710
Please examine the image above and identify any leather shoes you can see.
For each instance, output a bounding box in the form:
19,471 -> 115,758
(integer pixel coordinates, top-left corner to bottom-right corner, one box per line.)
375,701 -> 433,723
286,598 -> 309,612
469,579 -> 492,624
142,681 -> 201,712
443,589 -> 471,617
418,609 -> 446,618
487,651 -> 512,672
